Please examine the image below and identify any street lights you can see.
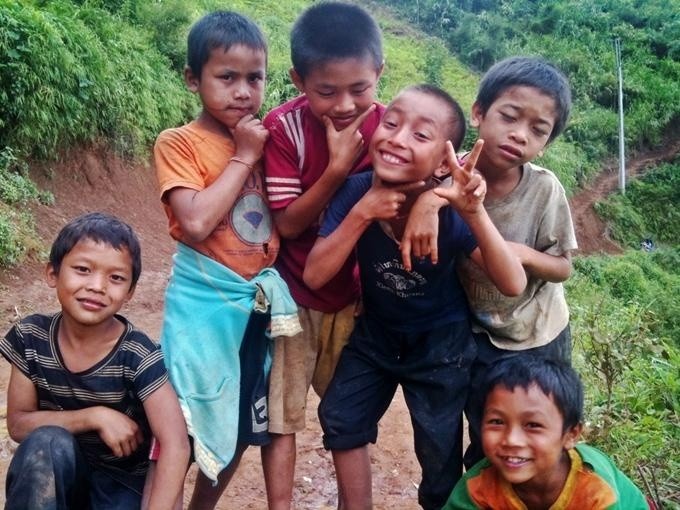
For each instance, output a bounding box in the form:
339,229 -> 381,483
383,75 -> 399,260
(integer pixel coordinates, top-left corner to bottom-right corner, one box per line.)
609,33 -> 626,197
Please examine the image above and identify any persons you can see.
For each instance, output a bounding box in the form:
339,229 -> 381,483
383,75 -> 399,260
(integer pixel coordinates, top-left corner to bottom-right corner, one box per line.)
442,347 -> 656,510
141,8 -> 282,509
650,239 -> 654,248
302,82 -> 529,510
431,55 -> 580,475
259,2 -> 389,509
1,210 -> 191,510
641,238 -> 652,251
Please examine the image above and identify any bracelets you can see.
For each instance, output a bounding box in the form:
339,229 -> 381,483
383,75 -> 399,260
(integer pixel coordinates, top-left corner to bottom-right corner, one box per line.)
228,154 -> 256,174
